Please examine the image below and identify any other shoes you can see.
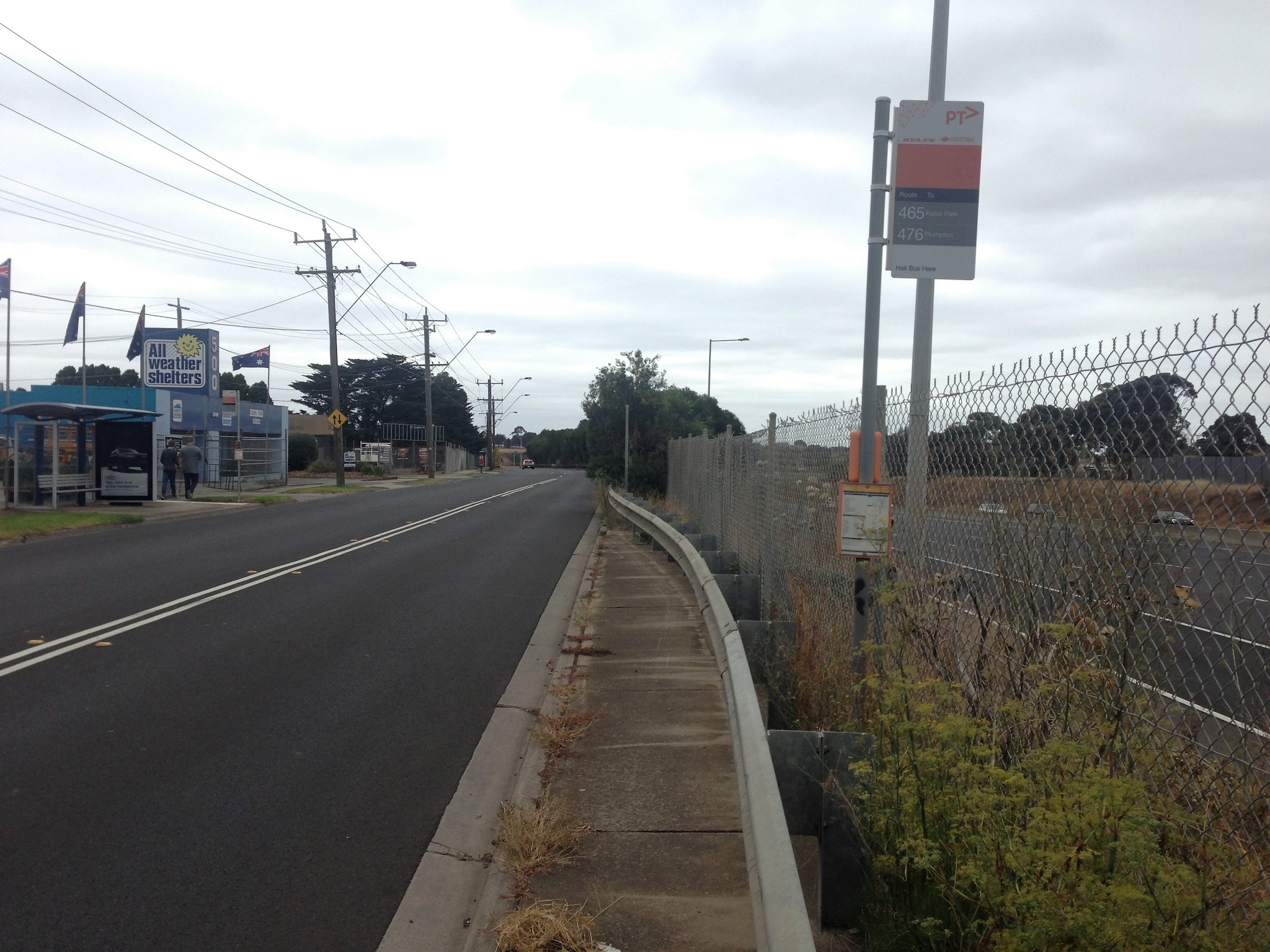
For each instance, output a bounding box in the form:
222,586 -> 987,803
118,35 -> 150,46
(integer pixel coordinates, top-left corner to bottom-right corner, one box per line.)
161,496 -> 165,500
186,490 -> 193,500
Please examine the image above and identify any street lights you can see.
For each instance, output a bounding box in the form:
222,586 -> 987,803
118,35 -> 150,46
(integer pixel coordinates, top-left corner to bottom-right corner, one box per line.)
707,338 -> 749,398
425,329 -> 496,478
492,376 -> 533,453
326,260 -> 417,486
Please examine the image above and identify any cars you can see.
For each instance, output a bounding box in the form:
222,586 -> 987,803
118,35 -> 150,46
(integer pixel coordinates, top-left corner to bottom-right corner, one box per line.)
824,500 -> 836,509
109,447 -> 147,460
1027,503 -> 1058,516
977,503 -> 1008,515
1152,511 -> 1196,526
522,460 -> 536,469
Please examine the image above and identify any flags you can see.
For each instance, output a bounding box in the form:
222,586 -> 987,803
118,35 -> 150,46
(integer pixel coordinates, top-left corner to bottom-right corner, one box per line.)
231,346 -> 269,371
126,307 -> 144,362
63,281 -> 85,347
0,258 -> 10,300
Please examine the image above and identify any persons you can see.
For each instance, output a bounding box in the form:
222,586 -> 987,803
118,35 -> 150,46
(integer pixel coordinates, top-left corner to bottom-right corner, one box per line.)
160,440 -> 178,501
178,437 -> 204,499
479,455 -> 484,473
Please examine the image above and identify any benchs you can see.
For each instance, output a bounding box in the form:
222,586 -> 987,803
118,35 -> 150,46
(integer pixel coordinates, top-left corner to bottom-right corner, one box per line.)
38,474 -> 104,493
